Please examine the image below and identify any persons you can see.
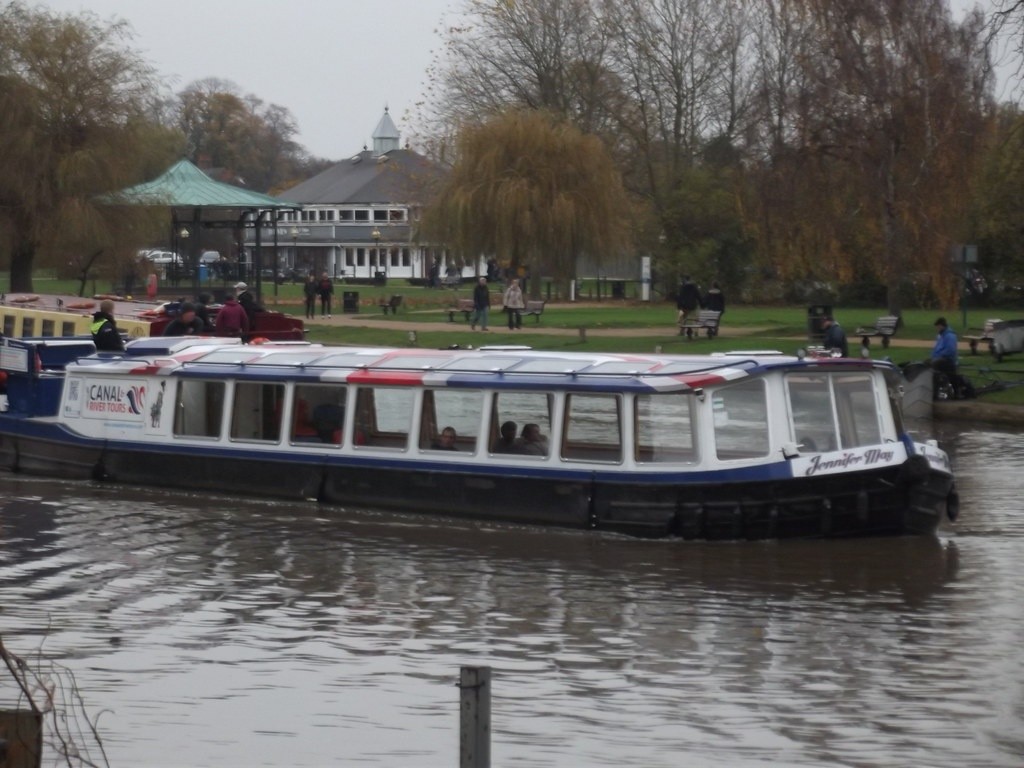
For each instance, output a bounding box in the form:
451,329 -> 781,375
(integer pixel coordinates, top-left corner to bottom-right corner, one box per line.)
194,291 -> 213,328
234,282 -> 254,332
705,279 -> 724,337
503,279 -> 524,329
931,317 -> 958,372
320,272 -> 334,319
519,423 -> 550,455
495,421 -> 521,455
678,275 -> 703,337
305,276 -> 320,319
820,318 -> 848,358
163,302 -> 203,337
472,277 -> 490,331
431,427 -> 458,451
92,299 -> 124,351
215,293 -> 249,336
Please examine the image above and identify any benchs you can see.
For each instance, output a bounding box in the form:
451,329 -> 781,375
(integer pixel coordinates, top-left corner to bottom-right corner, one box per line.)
963,319 -> 1004,355
379,294 -> 403,315
679,310 -> 723,340
855,317 -> 901,348
445,299 -> 475,323
512,301 -> 546,322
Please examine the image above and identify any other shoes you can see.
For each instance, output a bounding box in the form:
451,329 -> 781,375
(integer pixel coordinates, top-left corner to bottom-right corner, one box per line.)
321,316 -> 324,319
509,326 -> 513,330
481,328 -> 488,331
311,315 -> 315,319
306,315 -> 309,318
471,325 -> 475,330
515,325 -> 521,329
328,314 -> 331,318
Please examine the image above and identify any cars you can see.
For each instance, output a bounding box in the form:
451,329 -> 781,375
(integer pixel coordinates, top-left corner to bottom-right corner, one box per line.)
200,250 -> 221,265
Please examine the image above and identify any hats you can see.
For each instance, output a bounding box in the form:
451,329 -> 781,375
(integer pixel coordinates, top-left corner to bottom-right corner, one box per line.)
180,303 -> 196,315
935,318 -> 948,327
234,282 -> 247,290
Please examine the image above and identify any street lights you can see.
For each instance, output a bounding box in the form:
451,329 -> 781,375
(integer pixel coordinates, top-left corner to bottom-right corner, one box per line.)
371,226 -> 381,284
290,227 -> 300,269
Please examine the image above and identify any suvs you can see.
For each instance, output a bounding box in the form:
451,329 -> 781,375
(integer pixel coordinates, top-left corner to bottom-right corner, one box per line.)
148,251 -> 185,268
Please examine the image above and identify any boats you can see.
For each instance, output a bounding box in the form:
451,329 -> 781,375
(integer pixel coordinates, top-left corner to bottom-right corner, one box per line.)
0,332 -> 955,543
